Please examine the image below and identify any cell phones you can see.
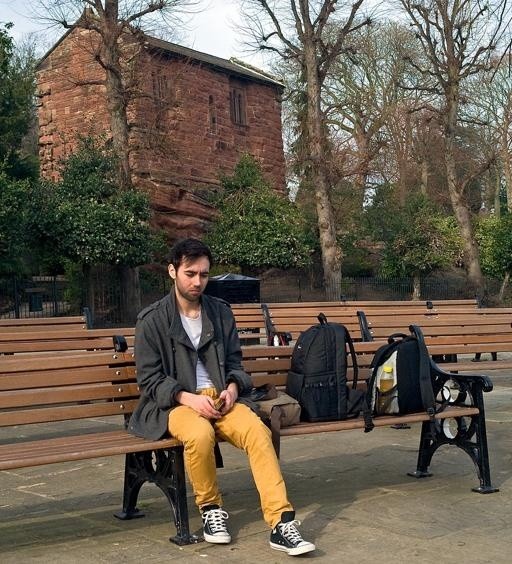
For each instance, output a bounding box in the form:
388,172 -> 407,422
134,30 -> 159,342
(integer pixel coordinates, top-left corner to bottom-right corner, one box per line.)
211,398 -> 224,410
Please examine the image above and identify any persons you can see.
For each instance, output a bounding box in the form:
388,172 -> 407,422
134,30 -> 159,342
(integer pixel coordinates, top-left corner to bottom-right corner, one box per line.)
126,238 -> 316,557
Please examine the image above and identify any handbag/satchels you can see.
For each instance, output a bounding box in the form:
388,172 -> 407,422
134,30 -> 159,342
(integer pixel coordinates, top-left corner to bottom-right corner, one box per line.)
245,382 -> 302,459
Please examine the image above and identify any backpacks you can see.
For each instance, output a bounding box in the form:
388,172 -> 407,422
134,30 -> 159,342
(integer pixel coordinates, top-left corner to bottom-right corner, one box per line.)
287,312 -> 374,433
368,325 -> 451,435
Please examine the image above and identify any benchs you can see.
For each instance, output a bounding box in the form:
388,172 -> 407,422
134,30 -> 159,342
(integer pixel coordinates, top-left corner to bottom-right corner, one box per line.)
0,308 -> 500,546
229,295 -> 512,390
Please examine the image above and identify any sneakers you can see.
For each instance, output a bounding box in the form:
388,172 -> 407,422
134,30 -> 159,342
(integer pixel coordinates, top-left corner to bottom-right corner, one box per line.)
200,504 -> 232,544
269,510 -> 316,557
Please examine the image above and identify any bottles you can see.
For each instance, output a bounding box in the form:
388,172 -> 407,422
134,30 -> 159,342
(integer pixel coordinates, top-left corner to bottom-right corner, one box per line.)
377,366 -> 394,412
